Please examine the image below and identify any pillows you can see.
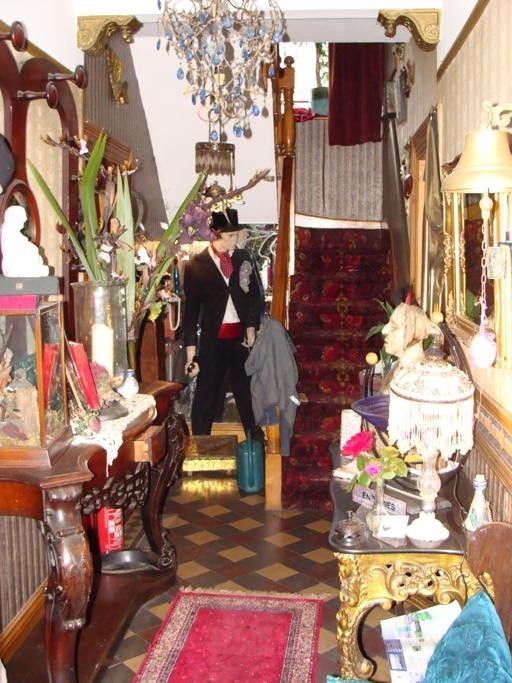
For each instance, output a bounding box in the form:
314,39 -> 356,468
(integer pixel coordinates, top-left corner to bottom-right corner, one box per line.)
424,592 -> 512,682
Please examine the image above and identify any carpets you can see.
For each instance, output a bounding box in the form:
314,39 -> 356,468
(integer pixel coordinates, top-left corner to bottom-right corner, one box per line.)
133,589 -> 326,682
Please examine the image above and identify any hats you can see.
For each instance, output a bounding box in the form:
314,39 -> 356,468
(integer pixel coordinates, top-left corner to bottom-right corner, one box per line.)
209,209 -> 244,233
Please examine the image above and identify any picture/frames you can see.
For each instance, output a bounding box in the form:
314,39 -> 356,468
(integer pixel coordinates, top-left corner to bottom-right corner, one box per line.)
442,153 -> 510,372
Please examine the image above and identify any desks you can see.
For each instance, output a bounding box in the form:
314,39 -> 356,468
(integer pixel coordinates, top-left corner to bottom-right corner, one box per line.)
328,439 -> 483,681
0,379 -> 190,681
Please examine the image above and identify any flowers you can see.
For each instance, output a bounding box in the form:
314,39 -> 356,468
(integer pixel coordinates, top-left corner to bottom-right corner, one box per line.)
342,432 -> 424,494
27,127 -> 273,371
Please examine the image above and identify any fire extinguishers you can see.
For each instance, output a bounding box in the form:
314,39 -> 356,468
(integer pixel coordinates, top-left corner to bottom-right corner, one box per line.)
83,486 -> 124,562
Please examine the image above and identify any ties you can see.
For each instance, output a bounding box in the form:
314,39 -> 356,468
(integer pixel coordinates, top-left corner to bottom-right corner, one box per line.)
211,242 -> 234,279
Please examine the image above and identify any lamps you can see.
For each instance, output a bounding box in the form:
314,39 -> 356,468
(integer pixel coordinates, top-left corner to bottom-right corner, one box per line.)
389,331 -> 476,547
440,102 -> 511,282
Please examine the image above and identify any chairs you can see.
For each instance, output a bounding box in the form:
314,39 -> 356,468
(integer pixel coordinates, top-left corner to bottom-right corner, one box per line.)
466,521 -> 512,650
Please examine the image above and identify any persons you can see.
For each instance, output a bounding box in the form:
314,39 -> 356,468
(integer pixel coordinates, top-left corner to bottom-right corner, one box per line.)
382,302 -> 431,356
182,207 -> 264,448
0,205 -> 52,277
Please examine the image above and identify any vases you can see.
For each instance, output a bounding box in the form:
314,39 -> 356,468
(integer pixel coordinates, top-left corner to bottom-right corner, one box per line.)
366,478 -> 390,534
